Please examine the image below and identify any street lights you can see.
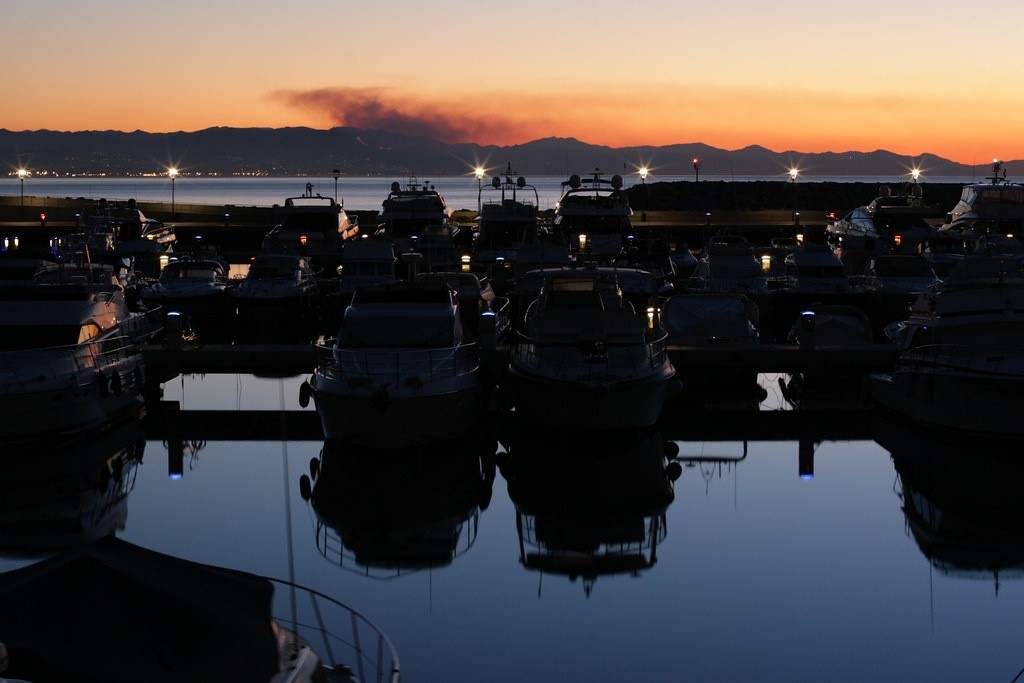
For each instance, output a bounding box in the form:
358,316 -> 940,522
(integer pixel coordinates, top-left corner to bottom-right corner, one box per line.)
693,159 -> 699,184
789,168 -> 799,220
639,166 -> 648,213
17,168 -> 29,217
333,168 -> 340,206
474,166 -> 484,213
167,166 -> 178,221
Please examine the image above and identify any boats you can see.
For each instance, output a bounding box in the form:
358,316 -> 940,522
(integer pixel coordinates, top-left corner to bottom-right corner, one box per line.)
297,434 -> 748,616
0,536 -> 405,682
0,156 -> 1024,518
872,429 -> 1023,633
